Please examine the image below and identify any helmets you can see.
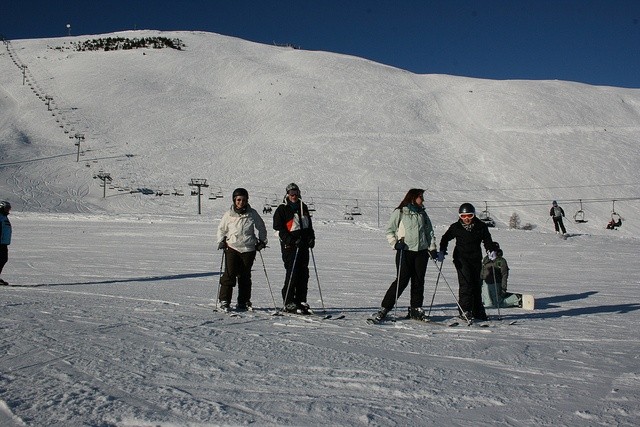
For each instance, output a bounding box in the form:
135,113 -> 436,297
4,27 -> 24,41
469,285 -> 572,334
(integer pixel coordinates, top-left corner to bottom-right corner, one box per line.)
459,203 -> 475,214
0,201 -> 11,209
233,188 -> 248,197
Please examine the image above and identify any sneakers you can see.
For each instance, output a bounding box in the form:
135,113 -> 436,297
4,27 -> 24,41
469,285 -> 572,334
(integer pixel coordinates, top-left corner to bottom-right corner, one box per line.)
299,302 -> 310,310
376,307 -> 389,321
238,301 -> 251,308
221,301 -> 230,309
411,307 -> 425,317
459,311 -> 473,318
0,279 -> 8,285
283,302 -> 297,311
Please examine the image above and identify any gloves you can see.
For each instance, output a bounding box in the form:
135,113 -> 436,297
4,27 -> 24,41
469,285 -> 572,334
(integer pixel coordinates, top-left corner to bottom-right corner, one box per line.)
490,251 -> 497,260
430,250 -> 439,263
501,289 -> 506,298
255,241 -> 265,251
217,240 -> 227,250
395,239 -> 407,250
295,239 -> 303,247
438,251 -> 448,262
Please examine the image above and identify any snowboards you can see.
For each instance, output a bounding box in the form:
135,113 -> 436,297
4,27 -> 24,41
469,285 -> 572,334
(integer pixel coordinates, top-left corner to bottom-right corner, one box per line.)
505,292 -> 534,311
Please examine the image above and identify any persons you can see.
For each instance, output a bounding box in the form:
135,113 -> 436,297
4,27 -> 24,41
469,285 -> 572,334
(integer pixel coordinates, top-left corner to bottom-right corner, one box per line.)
437,204 -> 493,325
272,183 -> 316,315
376,188 -> 437,321
215,188 -> 268,313
614,218 -> 622,229
481,242 -> 522,308
550,200 -> 568,236
0,201 -> 13,285
606,218 -> 615,229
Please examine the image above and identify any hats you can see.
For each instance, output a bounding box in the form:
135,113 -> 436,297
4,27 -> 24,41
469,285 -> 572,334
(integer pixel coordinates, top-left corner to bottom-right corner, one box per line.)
308,239 -> 315,248
283,183 -> 302,203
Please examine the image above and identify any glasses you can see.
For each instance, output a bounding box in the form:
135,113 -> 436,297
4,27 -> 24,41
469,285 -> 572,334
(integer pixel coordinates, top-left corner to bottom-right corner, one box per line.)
458,213 -> 474,220
287,190 -> 300,197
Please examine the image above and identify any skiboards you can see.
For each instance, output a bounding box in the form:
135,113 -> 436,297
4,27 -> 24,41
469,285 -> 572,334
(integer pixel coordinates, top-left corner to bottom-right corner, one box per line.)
197,303 -> 281,316
472,318 -> 518,327
269,307 -> 346,320
365,312 -> 459,327
